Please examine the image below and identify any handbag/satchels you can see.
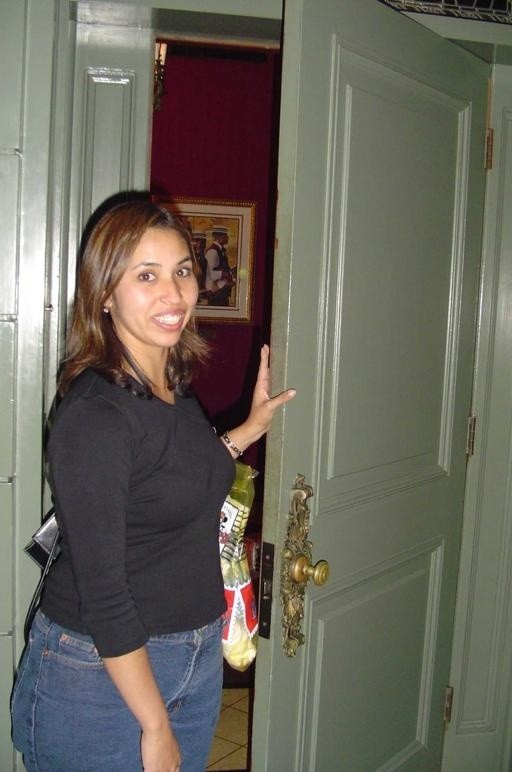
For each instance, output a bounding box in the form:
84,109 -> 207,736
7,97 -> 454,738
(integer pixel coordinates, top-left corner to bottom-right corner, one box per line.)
216,459 -> 261,675
22,506 -> 62,569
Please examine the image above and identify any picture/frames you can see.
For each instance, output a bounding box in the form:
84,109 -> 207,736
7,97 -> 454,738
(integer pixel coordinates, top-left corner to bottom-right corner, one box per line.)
156,196 -> 256,331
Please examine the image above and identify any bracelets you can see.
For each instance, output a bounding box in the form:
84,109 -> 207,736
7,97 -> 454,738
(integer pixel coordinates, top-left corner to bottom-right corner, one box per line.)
222,431 -> 245,457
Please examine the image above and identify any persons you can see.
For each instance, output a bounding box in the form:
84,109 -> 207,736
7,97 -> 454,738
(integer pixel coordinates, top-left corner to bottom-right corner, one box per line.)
200,226 -> 237,305
8,185 -> 298,772
